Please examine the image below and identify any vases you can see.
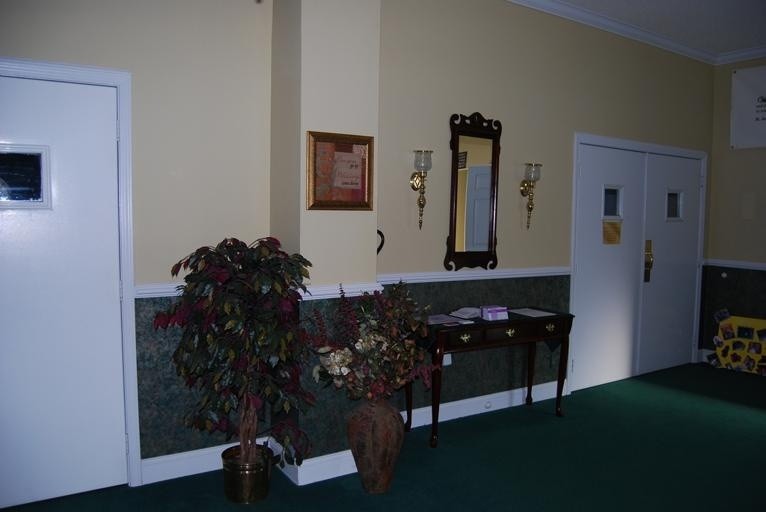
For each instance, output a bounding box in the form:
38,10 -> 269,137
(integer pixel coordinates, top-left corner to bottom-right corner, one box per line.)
345,402 -> 406,496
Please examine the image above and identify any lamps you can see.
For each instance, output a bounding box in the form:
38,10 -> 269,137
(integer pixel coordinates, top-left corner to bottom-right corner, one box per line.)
409,148 -> 433,232
519,160 -> 543,230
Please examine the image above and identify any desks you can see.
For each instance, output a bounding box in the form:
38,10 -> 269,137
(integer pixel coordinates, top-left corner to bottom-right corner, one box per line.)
405,305 -> 576,449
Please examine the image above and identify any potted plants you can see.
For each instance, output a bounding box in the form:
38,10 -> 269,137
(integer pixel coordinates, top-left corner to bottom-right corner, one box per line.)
152,235 -> 325,507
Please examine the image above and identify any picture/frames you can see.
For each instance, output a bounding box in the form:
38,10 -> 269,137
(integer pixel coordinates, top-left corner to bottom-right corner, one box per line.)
306,130 -> 375,214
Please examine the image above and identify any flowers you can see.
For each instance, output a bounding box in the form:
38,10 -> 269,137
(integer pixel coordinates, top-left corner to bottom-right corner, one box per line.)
312,275 -> 445,404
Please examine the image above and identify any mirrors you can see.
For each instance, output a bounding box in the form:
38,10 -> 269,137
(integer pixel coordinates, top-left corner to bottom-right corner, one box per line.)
443,111 -> 503,272
